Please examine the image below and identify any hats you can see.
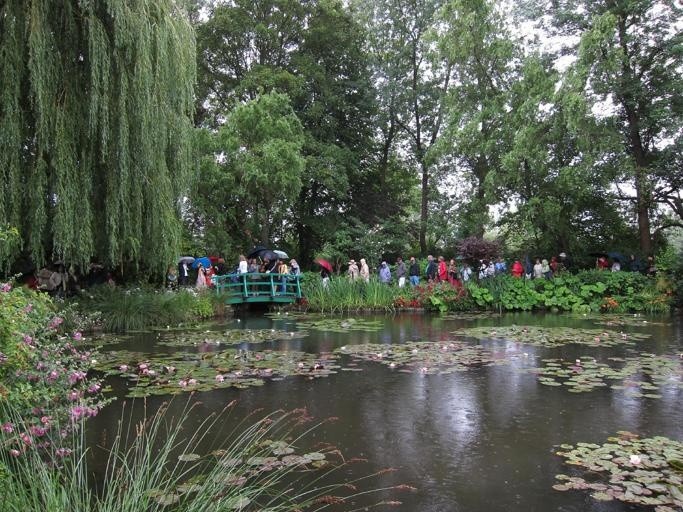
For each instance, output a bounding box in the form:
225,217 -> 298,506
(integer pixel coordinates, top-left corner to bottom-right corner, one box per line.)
559,252 -> 566,258
